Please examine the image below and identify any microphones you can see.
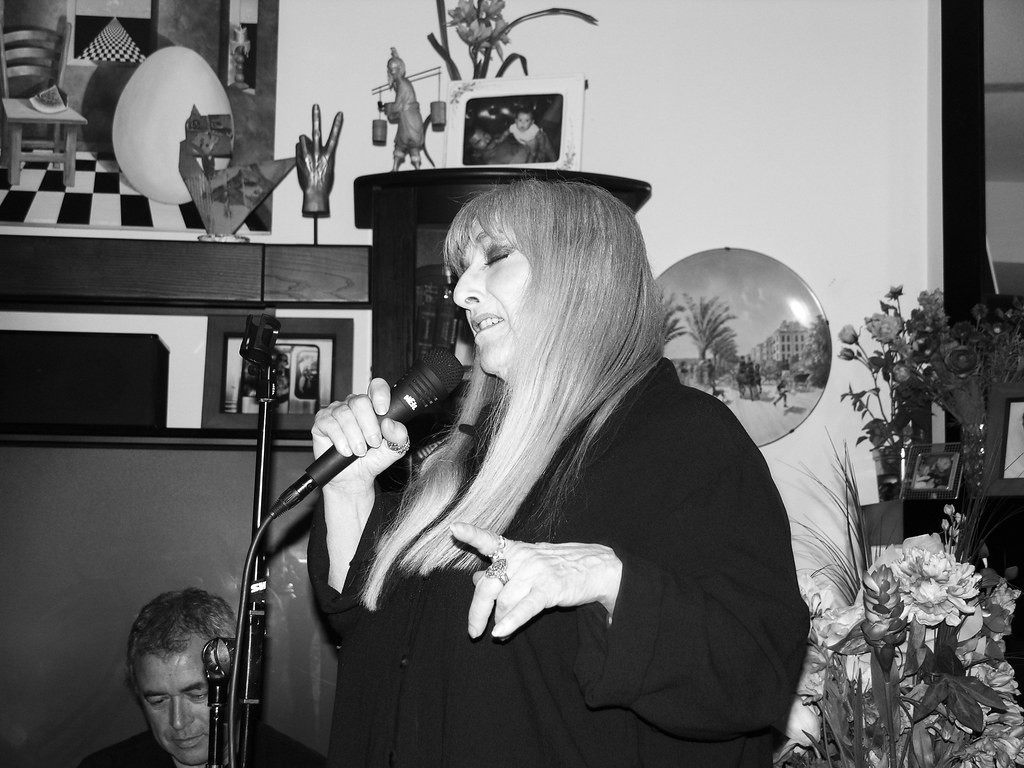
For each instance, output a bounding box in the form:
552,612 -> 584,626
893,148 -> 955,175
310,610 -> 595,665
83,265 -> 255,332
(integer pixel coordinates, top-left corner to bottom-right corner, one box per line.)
268,345 -> 465,516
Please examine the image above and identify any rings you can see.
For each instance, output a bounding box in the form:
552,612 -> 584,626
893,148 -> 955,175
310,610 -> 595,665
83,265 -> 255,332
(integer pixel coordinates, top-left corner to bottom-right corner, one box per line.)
484,533 -> 506,558
485,559 -> 510,586
387,435 -> 411,455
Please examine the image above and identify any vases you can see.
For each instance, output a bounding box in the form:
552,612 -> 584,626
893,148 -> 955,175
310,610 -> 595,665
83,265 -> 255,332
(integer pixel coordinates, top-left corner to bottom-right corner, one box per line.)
960,423 -> 986,501
869,447 -> 901,503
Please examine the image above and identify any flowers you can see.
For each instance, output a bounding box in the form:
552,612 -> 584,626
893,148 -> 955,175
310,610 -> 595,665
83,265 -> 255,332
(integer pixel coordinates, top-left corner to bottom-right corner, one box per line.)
427,0 -> 599,80
773,423 -> 1024,768
838,286 -> 938,447
864,289 -> 1024,424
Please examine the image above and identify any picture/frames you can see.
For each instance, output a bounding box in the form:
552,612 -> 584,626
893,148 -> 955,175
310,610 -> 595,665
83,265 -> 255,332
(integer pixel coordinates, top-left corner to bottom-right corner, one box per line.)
446,72 -> 585,171
899,442 -> 963,499
983,383 -> 1024,496
201,316 -> 356,429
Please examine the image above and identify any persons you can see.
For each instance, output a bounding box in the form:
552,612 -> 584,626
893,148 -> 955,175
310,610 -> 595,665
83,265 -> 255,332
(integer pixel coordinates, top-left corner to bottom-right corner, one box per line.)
679,354 -> 791,408
384,47 -> 425,172
913,457 -> 951,488
463,107 -> 556,164
77,588 -> 327,768
308,179 -> 810,768
